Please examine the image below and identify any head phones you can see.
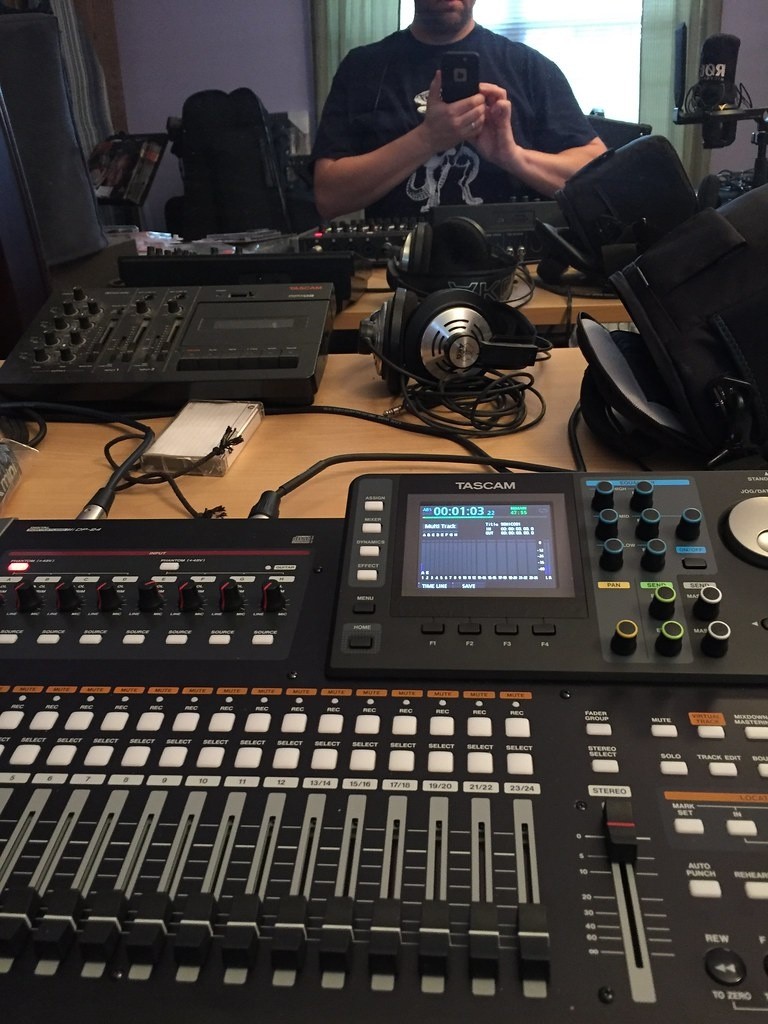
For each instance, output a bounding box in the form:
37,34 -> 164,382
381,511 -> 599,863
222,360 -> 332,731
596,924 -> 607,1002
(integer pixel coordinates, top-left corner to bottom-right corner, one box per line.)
356,288 -> 553,395
386,216 -> 535,309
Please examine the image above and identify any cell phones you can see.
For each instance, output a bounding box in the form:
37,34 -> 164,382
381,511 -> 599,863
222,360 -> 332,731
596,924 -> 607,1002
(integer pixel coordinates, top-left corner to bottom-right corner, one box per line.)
442,52 -> 480,105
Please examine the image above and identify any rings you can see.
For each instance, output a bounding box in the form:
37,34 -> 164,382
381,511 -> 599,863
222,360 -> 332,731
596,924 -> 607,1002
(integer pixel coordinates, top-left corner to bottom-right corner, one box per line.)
471,122 -> 476,129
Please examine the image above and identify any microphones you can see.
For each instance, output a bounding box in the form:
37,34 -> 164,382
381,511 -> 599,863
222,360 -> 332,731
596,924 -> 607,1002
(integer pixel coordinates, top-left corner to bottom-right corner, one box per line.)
698,33 -> 742,148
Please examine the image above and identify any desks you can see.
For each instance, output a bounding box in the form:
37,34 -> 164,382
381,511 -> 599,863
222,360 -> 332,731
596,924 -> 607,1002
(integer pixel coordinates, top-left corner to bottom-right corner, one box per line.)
329,257 -> 633,326
0,345 -> 591,520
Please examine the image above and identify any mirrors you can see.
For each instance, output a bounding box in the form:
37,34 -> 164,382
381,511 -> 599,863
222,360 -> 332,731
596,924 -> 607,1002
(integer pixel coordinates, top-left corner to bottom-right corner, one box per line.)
0,0 -> 724,289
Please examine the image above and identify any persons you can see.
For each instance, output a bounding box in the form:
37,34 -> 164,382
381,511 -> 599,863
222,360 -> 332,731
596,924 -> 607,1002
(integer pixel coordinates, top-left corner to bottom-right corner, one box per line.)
308,0 -> 607,218
